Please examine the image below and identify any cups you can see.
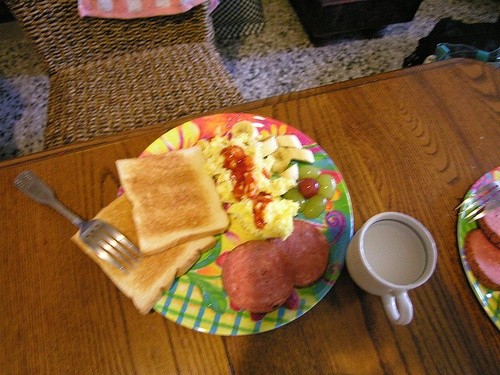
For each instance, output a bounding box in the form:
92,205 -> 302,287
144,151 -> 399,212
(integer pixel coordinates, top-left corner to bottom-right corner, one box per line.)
348,212 -> 437,325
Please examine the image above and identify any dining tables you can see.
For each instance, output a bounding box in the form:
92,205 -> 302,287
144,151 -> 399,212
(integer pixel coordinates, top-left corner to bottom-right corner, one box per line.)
0,58 -> 500,375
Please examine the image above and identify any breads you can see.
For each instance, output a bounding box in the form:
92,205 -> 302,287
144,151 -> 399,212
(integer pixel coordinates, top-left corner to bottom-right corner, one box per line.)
71,192 -> 217,314
115,145 -> 229,254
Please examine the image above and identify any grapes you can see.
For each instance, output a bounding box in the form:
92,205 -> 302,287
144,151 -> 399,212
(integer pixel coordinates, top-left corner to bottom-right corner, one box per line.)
283,164 -> 336,217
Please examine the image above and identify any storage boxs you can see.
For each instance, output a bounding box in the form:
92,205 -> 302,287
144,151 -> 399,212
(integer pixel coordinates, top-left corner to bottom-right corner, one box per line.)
288,0 -> 423,38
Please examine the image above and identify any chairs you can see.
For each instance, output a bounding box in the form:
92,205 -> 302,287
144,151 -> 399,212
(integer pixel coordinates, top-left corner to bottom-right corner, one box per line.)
4,0 -> 247,152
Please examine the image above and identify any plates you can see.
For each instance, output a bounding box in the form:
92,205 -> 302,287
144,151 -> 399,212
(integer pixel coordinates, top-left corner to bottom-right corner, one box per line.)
118,113 -> 354,336
458,166 -> 500,330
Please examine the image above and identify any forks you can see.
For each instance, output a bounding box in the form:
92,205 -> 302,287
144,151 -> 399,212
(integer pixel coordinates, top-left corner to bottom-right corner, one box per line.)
454,180 -> 500,223
12,169 -> 142,275
